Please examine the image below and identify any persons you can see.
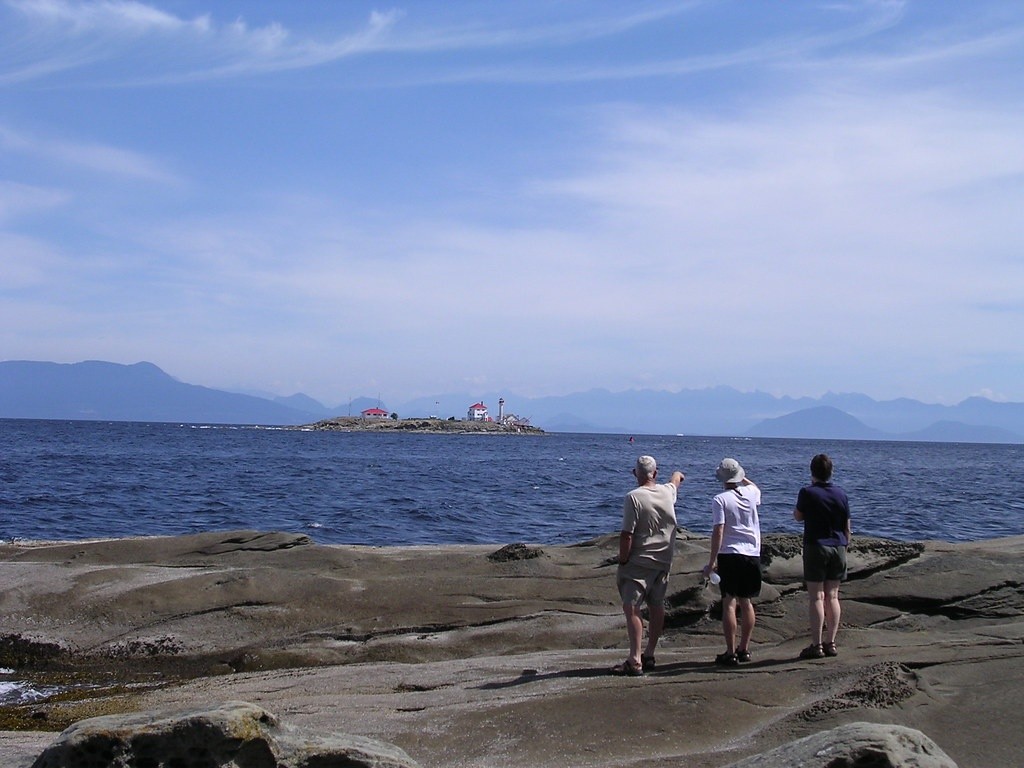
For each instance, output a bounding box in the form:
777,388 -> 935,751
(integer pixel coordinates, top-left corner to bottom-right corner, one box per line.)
705,457 -> 762,665
610,456 -> 684,676
795,454 -> 851,658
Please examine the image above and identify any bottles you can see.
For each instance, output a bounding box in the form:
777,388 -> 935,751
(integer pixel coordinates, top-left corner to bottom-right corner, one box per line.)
704,565 -> 721,585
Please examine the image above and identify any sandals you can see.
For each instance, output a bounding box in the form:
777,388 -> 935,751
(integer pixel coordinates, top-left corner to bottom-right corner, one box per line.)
822,641 -> 838,656
715,651 -> 739,665
736,645 -> 752,662
800,644 -> 825,659
609,660 -> 644,676
640,653 -> 656,672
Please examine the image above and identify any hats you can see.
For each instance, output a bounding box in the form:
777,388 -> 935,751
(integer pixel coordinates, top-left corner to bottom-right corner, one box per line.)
716,457 -> 746,483
